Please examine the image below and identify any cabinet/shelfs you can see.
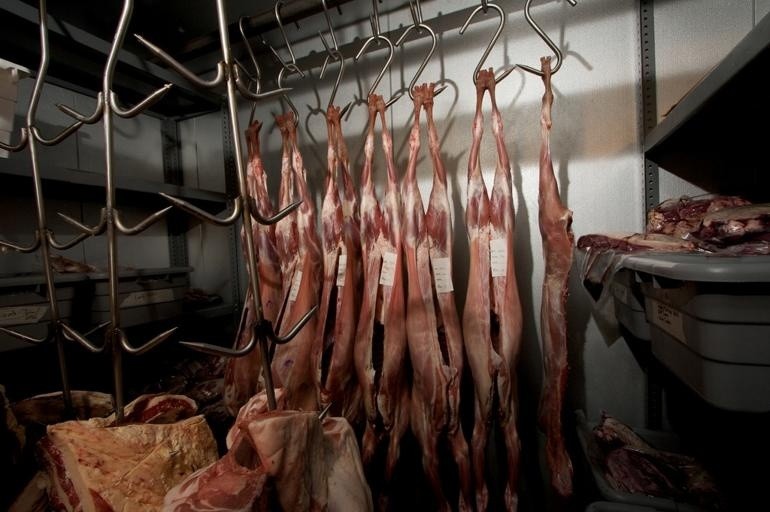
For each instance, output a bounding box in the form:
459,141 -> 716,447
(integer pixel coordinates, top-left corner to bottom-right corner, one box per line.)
0,0 -> 236,399
